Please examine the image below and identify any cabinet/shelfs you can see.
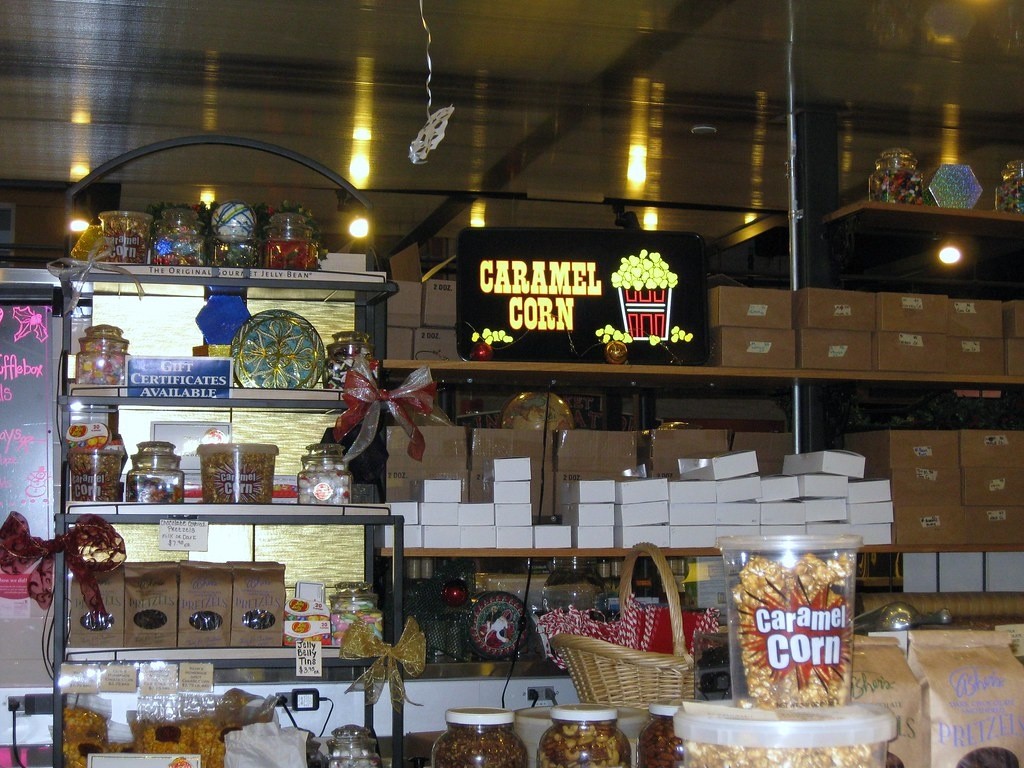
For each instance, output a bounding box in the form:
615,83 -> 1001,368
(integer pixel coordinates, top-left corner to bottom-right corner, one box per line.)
50,133 -> 404,768
373,201 -> 1024,558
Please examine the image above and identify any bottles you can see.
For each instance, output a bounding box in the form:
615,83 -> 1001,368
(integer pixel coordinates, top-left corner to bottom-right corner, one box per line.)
297,444 -> 353,505
125,442 -> 185,504
322,330 -> 373,389
292,727 -> 326,768
995,161 -> 1024,214
537,704 -> 632,768
541,557 -> 609,621
98,206 -> 318,271
869,148 -> 923,206
637,699 -> 705,768
75,324 -> 129,386
596,558 -> 659,623
324,724 -> 379,768
329,583 -> 384,648
431,708 -> 528,768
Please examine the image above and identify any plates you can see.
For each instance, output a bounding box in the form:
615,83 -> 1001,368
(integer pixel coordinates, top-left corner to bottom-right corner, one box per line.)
459,591 -> 531,660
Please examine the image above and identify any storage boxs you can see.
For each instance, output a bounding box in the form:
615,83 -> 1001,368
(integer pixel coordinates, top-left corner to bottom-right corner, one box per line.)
707,284 -> 1022,376
476,573 -> 550,611
377,427 -> 1024,550
385,243 -> 457,360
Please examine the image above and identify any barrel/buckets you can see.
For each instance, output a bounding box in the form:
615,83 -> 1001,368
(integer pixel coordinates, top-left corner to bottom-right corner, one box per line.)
513,706 -> 649,768
672,698 -> 898,768
68,450 -> 124,502
715,535 -> 866,714
197,443 -> 280,504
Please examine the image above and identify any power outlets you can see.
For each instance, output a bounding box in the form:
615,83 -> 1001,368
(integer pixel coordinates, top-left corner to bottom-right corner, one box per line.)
8,696 -> 25,711
527,686 -> 554,701
275,692 -> 292,707
